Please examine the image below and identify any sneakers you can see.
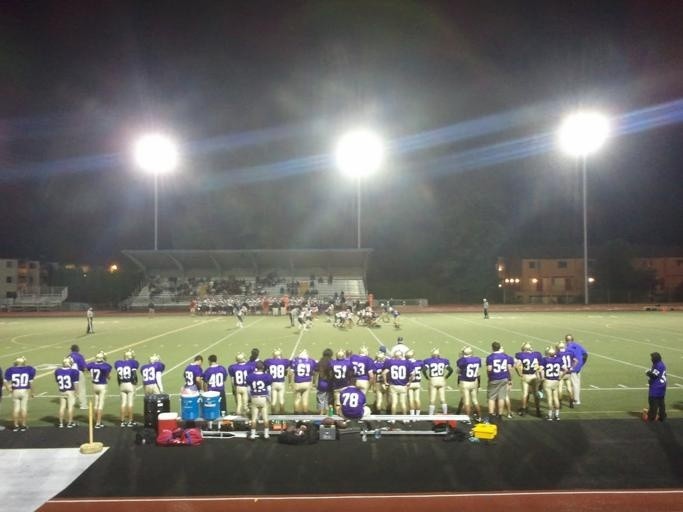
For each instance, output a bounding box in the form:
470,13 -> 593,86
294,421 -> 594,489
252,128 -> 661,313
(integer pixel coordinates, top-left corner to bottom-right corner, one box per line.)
94,423 -> 105,429
12,425 -> 28,432
120,421 -> 137,428
573,401 -> 581,405
547,415 -> 553,421
507,414 -> 512,419
56,423 -> 64,429
555,415 -> 560,420
67,421 -> 80,429
475,417 -> 482,423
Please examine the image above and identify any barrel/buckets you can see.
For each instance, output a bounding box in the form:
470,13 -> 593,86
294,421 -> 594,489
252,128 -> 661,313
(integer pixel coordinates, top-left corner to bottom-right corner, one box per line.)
201,391 -> 220,420
145,393 -> 170,427
180,391 -> 199,421
156,411 -> 177,435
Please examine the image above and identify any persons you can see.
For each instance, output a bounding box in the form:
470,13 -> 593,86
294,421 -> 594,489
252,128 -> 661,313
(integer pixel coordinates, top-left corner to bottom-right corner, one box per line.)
184,348 -> 317,439
481,297 -> 490,320
0,366 -> 5,430
316,337 -> 453,425
456,334 -> 586,423
55,345 -> 165,429
645,351 -> 667,422
2,356 -> 36,432
84,306 -> 95,334
143,269 -> 401,330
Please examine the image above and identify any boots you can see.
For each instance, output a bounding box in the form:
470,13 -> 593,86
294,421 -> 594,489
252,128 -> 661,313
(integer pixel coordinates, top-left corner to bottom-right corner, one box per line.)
570,399 -> 575,409
559,399 -> 563,409
263,427 -> 271,440
248,428 -> 257,440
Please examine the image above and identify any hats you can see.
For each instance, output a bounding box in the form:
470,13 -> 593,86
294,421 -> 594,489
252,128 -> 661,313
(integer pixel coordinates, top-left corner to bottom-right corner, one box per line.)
558,342 -> 567,352
398,336 -> 404,344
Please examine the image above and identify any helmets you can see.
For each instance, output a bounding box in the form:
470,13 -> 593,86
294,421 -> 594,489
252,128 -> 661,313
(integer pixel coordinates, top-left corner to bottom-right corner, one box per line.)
565,335 -> 573,343
14,357 -> 26,366
235,353 -> 246,363
95,352 -> 107,363
545,346 -> 556,356
272,348 -> 282,359
335,345 -> 440,361
123,350 -> 136,361
463,346 -> 472,357
520,341 -> 532,352
62,357 -> 74,368
149,355 -> 160,363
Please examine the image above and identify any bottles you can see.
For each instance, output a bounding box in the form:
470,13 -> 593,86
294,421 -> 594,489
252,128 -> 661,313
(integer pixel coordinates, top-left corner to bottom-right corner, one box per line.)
361,425 -> 368,442
374,424 -> 381,439
329,404 -> 334,417
484,416 -> 490,424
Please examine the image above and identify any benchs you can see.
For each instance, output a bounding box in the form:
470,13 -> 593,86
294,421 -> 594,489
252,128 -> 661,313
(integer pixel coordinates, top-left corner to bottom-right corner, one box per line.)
0,286 -> 68,312
130,276 -> 368,306
176,414 -> 472,439
